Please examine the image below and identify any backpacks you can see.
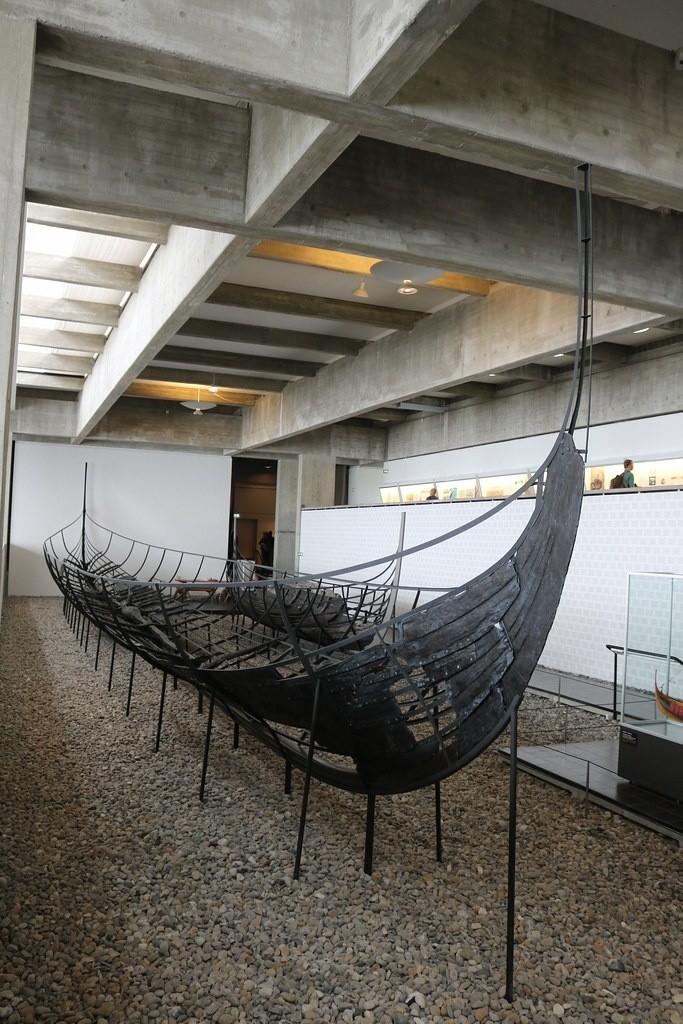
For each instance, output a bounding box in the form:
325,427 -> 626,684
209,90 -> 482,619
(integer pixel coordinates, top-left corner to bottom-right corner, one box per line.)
610,471 -> 629,489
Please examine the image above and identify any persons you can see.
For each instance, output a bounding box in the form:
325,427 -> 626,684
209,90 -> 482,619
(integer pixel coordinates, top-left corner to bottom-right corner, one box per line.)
620,460 -> 637,488
259,530 -> 274,574
426,489 -> 439,500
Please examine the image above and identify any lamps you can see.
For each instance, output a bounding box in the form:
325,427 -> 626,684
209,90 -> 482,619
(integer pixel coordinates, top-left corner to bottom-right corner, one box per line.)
180,386 -> 216,415
371,260 -> 444,295
